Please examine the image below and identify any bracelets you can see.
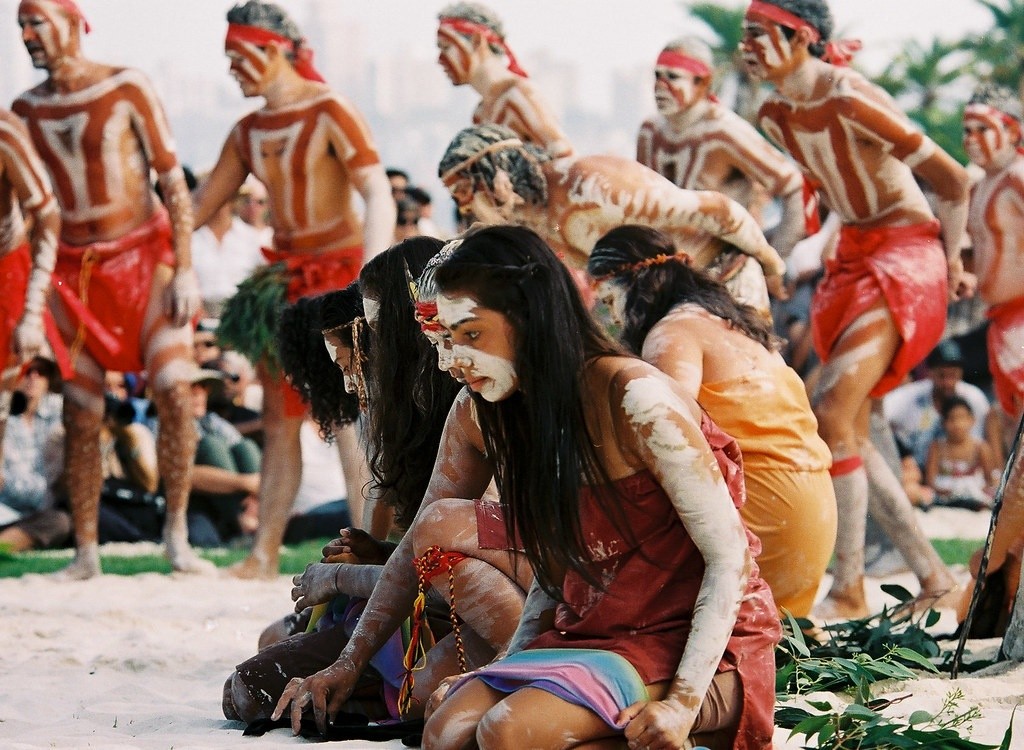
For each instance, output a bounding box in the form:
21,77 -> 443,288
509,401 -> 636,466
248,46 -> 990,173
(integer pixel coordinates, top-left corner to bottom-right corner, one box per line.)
329,563 -> 346,595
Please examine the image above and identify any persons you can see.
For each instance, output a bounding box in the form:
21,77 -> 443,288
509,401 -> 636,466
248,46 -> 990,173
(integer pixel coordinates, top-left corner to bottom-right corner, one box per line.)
221,221 -> 783,750
0,0 -> 1024,641
